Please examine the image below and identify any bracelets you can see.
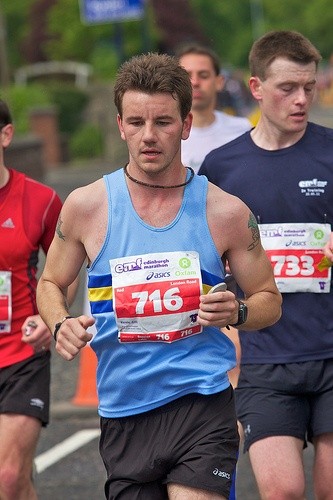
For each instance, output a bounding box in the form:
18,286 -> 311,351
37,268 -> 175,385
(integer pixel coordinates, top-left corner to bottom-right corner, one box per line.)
53,313 -> 72,342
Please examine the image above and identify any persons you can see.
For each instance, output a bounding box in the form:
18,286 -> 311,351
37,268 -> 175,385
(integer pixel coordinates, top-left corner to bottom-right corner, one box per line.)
177,46 -> 255,174
0,100 -> 80,500
195,29 -> 332,500
36,53 -> 282,500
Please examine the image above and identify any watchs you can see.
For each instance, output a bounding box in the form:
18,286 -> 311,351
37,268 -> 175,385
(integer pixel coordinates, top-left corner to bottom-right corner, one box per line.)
225,298 -> 249,330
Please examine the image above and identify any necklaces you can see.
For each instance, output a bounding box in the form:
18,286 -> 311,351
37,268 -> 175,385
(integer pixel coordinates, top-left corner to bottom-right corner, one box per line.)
122,161 -> 197,190
